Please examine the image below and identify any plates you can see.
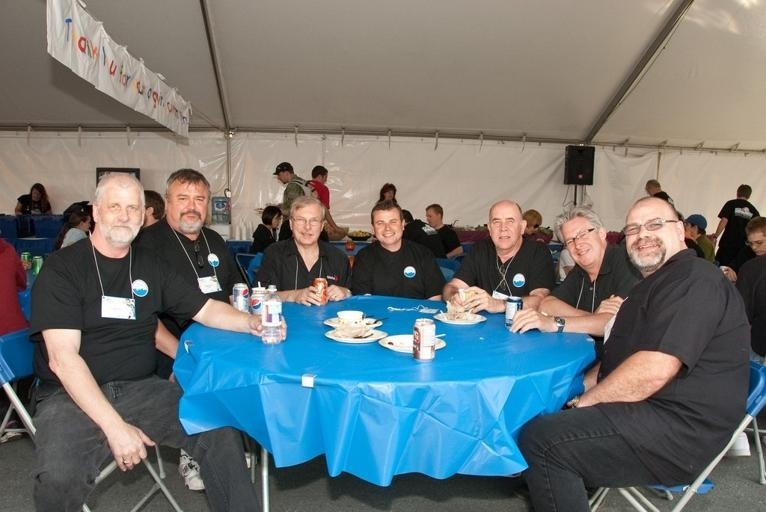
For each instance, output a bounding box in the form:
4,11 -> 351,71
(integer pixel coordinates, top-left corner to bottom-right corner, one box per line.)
325,329 -> 387,342
434,313 -> 488,325
324,317 -> 381,331
379,335 -> 446,354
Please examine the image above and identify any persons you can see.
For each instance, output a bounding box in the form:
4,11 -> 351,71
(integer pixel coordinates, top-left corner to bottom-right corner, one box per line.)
0,235 -> 42,446
15,182 -> 52,217
343,201 -> 447,302
135,168 -> 256,492
440,199 -> 556,319
506,205 -> 650,369
251,194 -> 351,308
513,196 -> 753,511
23,172 -> 288,511
724,253 -> 765,459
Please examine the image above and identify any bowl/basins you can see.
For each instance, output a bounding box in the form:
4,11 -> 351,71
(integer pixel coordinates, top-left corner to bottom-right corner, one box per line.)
327,231 -> 372,241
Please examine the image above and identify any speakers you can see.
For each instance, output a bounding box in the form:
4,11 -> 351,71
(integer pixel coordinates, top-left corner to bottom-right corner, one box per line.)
564,145 -> 595,185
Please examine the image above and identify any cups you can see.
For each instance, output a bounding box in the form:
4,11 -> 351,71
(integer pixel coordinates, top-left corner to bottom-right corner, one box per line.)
338,309 -> 364,327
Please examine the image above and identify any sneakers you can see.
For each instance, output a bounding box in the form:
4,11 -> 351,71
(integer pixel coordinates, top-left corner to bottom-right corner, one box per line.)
174,455 -> 207,493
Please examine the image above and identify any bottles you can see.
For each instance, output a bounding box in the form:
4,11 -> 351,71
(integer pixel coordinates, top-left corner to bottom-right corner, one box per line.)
345,239 -> 354,251
454,222 -> 487,233
259,285 -> 285,346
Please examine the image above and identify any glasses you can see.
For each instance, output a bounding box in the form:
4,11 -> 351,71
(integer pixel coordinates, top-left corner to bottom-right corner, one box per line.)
745,240 -> 766,246
290,216 -> 324,226
564,228 -> 595,248
623,218 -> 678,236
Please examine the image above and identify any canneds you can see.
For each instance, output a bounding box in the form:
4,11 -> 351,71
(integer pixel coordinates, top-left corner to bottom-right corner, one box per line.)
505,296 -> 523,327
413,318 -> 435,361
313,278 -> 328,305
32,256 -> 44,276
21,252 -> 32,270
250,294 -> 265,316
233,282 -> 250,313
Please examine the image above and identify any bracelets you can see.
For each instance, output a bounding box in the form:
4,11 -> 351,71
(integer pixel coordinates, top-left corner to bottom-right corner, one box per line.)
557,317 -> 567,334
567,392 -> 585,408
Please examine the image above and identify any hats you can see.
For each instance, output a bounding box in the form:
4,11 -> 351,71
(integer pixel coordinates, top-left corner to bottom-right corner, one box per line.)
682,214 -> 708,231
272,161 -> 294,176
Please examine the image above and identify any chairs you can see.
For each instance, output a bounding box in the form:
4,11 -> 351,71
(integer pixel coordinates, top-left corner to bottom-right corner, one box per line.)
223,229 -> 569,295
0,323 -> 194,511
0,210 -> 70,445
582,347 -> 766,512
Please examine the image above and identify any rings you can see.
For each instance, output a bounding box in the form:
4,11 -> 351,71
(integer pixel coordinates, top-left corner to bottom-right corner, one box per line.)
123,457 -> 132,466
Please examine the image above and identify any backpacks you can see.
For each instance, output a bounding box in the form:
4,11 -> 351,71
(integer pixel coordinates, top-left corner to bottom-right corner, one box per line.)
291,178 -> 320,198
